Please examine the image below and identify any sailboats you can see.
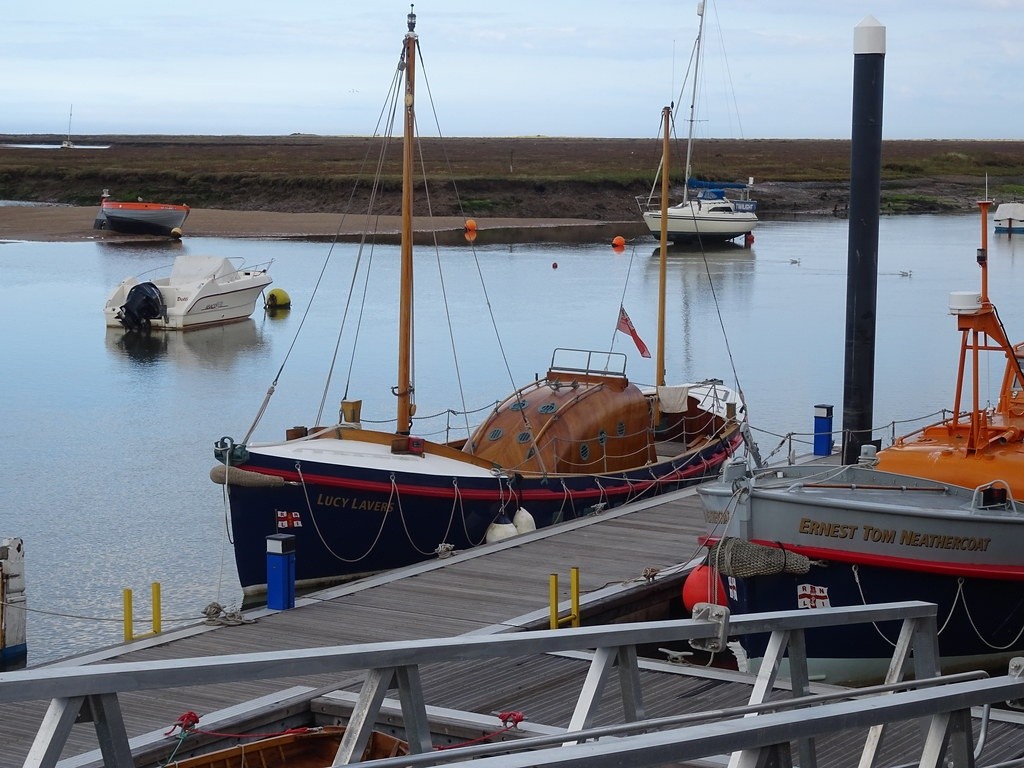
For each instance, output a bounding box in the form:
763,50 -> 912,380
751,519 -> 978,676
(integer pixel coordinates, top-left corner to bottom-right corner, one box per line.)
208,0 -> 747,596
60,103 -> 74,149
635,1 -> 758,243
643,239 -> 755,383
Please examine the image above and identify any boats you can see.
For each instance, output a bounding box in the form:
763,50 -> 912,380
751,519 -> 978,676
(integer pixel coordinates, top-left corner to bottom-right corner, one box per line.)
679,174 -> 1022,684
992,191 -> 1024,235
94,189 -> 190,238
105,318 -> 263,371
100,256 -> 277,330
